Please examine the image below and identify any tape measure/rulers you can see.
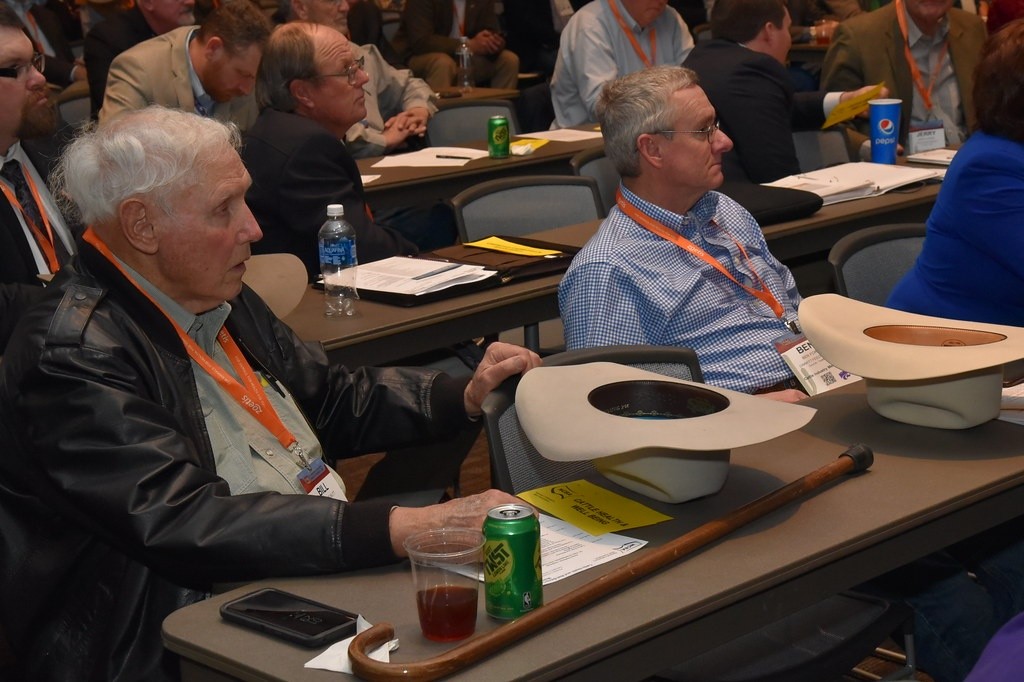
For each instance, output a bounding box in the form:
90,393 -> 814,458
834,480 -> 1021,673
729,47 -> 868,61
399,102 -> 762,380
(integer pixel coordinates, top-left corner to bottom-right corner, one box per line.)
411,264 -> 462,280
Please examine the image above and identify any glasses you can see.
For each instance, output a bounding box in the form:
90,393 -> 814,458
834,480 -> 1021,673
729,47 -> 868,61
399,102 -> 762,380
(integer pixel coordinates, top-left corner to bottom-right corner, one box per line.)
318,57 -> 365,85
634,121 -> 719,152
0,53 -> 45,81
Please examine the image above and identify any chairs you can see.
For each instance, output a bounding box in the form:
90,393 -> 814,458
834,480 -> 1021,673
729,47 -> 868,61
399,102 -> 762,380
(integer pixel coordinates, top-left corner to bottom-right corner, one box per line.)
57,16 -> 927,682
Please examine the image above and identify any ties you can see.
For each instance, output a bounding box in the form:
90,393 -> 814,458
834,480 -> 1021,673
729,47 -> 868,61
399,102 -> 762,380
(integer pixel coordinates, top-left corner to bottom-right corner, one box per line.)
1,159 -> 71,274
193,94 -> 214,116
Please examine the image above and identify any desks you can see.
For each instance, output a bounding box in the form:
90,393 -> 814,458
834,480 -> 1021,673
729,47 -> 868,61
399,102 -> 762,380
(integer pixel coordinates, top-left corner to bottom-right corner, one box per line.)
353,122 -> 604,214
277,143 -> 965,370
158,377 -> 1024,682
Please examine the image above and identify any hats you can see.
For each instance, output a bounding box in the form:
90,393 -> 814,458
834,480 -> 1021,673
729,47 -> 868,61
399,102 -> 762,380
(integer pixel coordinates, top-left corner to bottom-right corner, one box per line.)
518,362 -> 819,501
797,293 -> 1024,429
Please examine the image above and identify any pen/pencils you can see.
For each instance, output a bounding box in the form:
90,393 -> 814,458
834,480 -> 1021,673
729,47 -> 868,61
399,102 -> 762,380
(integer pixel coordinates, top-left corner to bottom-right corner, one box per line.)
435,155 -> 472,159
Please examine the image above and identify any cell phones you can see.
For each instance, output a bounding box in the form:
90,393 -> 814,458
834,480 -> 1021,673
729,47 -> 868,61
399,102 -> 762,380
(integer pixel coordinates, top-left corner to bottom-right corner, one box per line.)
219,587 -> 358,648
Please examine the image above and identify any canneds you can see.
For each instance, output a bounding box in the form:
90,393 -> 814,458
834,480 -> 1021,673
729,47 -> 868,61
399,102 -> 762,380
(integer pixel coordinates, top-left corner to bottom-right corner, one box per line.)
487,115 -> 509,159
482,503 -> 544,621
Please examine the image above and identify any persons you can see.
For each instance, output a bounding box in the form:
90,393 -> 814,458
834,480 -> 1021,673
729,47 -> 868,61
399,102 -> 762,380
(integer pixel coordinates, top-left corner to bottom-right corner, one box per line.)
558,66 -> 1023,681
0,105 -> 544,682
508,0 -> 1024,189
886,19 -> 1024,330
0,0 -> 518,501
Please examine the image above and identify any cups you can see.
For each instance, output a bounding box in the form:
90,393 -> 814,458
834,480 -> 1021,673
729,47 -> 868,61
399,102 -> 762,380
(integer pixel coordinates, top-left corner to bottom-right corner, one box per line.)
402,526 -> 488,645
867,98 -> 903,165
813,18 -> 833,46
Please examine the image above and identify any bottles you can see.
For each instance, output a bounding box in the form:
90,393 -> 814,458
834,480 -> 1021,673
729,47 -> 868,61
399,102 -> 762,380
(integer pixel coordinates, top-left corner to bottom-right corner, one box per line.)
319,203 -> 358,318
455,36 -> 476,94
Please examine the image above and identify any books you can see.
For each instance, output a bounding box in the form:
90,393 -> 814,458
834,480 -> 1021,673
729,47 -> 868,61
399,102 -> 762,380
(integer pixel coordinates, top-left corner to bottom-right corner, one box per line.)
767,162 -> 939,206
